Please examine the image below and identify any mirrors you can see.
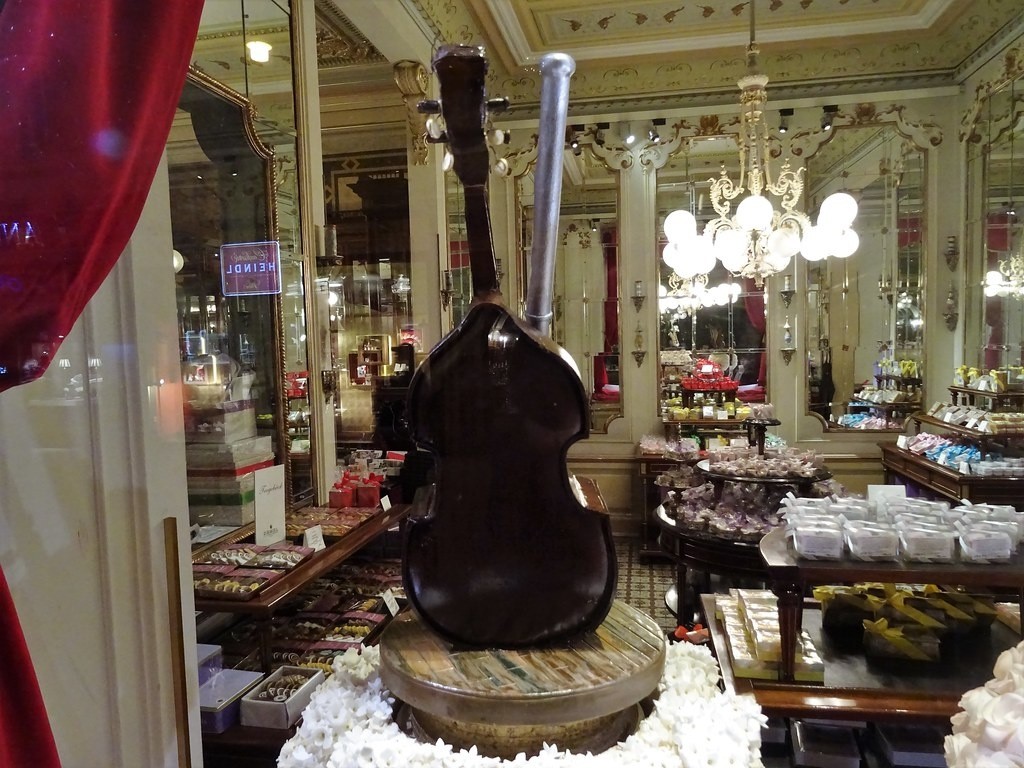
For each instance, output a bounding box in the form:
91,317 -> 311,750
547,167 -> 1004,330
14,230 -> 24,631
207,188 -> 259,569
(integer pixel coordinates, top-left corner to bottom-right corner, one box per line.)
805,121 -> 928,434
165,65 -> 294,561
657,137 -> 770,407
504,129 -> 634,433
961,71 -> 1024,379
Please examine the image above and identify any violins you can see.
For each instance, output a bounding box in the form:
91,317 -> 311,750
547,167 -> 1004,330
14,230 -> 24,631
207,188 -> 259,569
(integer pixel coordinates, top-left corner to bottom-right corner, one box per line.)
392,48 -> 619,650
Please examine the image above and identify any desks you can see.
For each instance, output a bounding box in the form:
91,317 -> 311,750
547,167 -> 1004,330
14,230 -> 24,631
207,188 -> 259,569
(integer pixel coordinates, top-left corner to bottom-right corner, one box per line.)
700,593 -> 1024,725
759,525 -> 1024,685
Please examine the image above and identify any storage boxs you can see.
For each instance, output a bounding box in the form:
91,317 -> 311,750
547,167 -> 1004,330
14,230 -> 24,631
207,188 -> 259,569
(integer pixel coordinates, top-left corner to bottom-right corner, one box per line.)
183,434 -> 276,478
184,400 -> 257,445
197,644 -> 326,734
187,478 -> 254,527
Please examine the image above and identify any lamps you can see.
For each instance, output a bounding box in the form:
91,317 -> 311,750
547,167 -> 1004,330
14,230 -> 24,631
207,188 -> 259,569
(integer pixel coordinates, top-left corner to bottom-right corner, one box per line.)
648,129 -> 662,141
247,41 -> 272,62
985,247 -> 1024,297
779,275 -> 795,307
663,0 -> 860,291
631,280 -> 646,314
942,280 -> 959,330
820,118 -> 831,130
631,320 -> 647,368
658,152 -> 743,318
569,137 -> 578,147
943,236 -> 960,272
778,118 -> 788,133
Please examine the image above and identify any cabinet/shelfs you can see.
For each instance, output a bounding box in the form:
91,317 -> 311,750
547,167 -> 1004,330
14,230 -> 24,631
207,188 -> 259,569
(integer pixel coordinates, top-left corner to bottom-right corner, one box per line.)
877,387 -> 1024,507
653,456 -> 861,625
827,375 -> 922,429
191,499 -> 415,754
